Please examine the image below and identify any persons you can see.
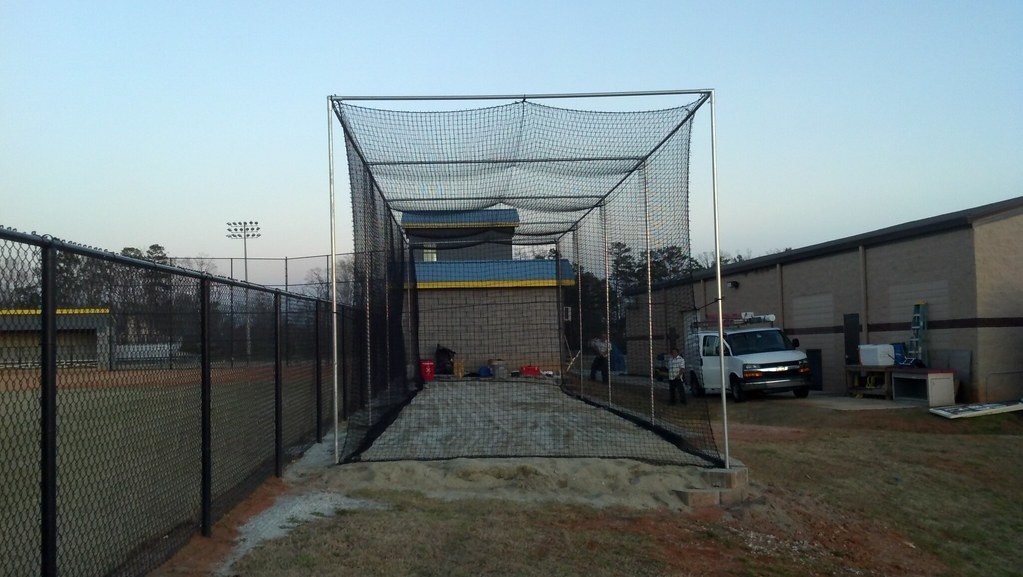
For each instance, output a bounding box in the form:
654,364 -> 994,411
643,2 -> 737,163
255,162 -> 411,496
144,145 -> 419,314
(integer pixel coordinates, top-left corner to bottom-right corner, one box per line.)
667,348 -> 688,406
589,331 -> 612,384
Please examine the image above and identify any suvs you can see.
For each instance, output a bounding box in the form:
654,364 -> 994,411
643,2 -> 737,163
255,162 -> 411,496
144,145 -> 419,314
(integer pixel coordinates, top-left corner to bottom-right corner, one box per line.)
683,325 -> 813,404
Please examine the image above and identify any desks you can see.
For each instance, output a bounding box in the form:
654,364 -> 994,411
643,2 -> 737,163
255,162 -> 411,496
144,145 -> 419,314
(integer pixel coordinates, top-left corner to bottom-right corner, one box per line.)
844,365 -> 893,399
891,368 -> 960,407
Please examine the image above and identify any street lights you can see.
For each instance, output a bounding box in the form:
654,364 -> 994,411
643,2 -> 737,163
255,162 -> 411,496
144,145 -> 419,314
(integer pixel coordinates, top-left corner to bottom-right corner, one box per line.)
224,221 -> 261,358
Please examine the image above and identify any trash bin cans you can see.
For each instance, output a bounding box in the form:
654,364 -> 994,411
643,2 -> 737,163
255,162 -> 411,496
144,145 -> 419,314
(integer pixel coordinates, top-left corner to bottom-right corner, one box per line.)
421,359 -> 435,380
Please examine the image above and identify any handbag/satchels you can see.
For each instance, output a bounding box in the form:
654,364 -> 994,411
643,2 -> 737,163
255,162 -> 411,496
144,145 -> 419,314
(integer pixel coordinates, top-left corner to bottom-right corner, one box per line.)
432,344 -> 457,374
